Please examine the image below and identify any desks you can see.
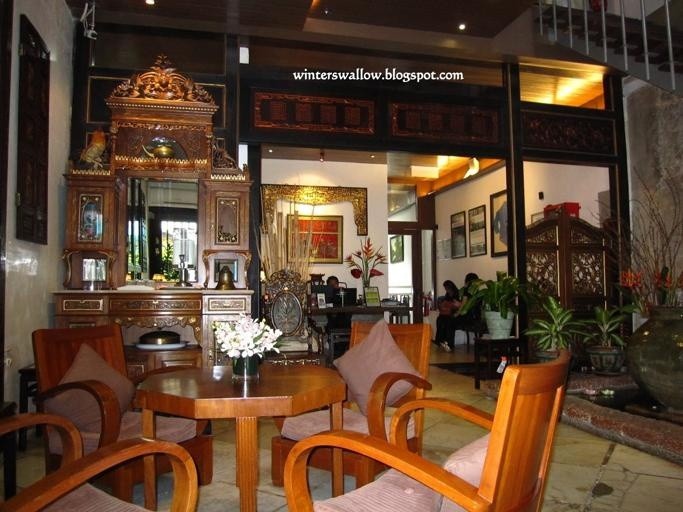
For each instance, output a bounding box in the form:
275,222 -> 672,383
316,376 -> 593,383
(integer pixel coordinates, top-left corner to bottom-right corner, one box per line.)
313,301 -> 413,365
473,337 -> 522,392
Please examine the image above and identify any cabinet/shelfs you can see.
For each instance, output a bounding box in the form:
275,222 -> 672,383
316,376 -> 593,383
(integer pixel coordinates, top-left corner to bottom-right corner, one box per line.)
204,180 -> 247,250
53,292 -> 254,365
62,173 -> 114,253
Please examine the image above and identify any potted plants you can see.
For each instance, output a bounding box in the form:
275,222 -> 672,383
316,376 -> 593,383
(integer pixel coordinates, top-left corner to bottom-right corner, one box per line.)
158,241 -> 179,284
523,295 -> 598,366
452,268 -> 552,338
580,306 -> 628,369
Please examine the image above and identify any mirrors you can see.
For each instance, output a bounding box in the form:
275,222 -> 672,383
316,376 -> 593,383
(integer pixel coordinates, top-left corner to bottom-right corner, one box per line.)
120,169 -> 204,286
80,257 -> 106,282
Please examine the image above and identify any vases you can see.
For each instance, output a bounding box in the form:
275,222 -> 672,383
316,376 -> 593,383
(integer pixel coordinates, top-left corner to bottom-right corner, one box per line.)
361,279 -> 370,302
231,355 -> 260,382
625,309 -> 682,412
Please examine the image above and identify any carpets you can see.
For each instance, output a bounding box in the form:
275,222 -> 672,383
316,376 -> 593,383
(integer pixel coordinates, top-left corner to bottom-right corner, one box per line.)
424,360 -> 508,381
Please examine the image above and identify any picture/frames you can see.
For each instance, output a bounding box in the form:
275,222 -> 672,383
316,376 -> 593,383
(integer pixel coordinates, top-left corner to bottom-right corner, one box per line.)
489,190 -> 509,257
285,214 -> 342,264
15,14 -> 49,245
467,205 -> 487,257
450,211 -> 466,258
389,234 -> 403,264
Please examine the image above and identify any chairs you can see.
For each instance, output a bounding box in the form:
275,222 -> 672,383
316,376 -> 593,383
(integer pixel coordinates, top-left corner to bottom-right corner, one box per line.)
0,412 -> 197,512
388,293 -> 411,325
280,347 -> 571,512
28,322 -> 216,502
267,318 -> 434,491
259,267 -> 323,364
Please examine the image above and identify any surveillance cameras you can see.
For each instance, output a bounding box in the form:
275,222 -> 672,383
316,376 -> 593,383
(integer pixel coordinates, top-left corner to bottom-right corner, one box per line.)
83,29 -> 98,40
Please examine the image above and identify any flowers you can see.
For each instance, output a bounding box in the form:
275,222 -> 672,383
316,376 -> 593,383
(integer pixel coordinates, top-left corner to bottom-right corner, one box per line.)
344,237 -> 389,286
588,162 -> 683,308
207,313 -> 284,354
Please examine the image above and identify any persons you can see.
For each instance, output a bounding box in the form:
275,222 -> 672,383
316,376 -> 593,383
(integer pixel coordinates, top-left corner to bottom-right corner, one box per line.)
435,280 -> 458,345
327,276 -> 339,288
440,273 -> 479,352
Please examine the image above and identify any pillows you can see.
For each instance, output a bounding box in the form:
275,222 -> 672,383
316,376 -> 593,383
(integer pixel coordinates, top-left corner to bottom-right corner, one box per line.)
328,318 -> 419,420
45,342 -> 137,432
439,428 -> 491,512
